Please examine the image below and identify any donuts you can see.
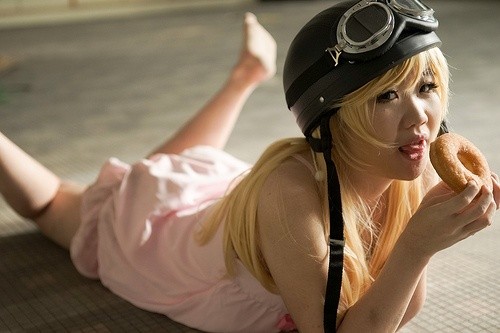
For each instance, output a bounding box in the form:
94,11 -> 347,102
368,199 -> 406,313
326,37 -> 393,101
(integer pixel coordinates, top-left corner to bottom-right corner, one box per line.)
430,133 -> 493,199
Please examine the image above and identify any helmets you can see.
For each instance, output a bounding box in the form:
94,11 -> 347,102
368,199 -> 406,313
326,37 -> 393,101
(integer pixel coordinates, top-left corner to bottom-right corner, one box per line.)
282,3 -> 442,137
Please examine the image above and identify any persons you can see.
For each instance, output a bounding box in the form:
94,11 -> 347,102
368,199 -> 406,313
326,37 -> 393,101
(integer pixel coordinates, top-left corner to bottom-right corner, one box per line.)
0,0 -> 500,333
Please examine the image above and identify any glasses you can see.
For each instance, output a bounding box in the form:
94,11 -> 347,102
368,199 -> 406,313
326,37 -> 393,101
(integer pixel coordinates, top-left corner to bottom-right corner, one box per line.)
324,0 -> 439,69
